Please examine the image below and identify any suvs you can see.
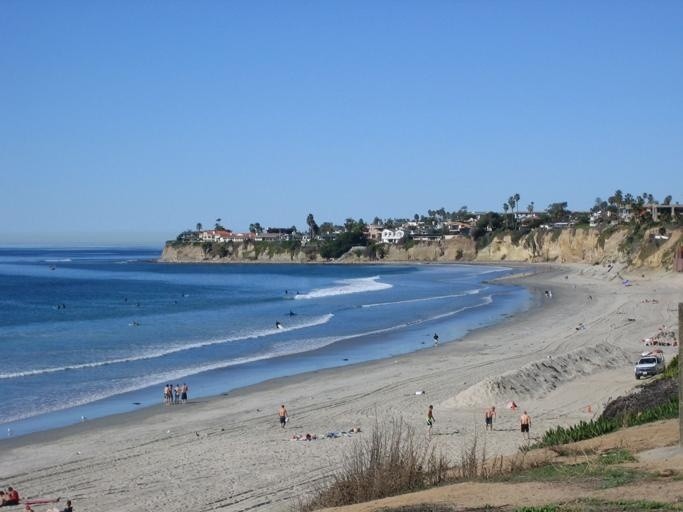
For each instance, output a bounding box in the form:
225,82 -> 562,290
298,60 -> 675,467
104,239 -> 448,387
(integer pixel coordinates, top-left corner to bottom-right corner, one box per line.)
634,356 -> 665,379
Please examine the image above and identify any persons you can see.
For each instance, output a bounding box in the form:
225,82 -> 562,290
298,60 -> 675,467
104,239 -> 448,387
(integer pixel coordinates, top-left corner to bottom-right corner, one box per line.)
433,333 -> 439,347
63,500 -> 73,512
278,404 -> 289,431
6,486 -> 19,505
484,406 -> 496,432
0,491 -> 11,507
426,404 -> 436,438
25,506 -> 33,512
163,383 -> 188,408
519,410 -> 531,440
